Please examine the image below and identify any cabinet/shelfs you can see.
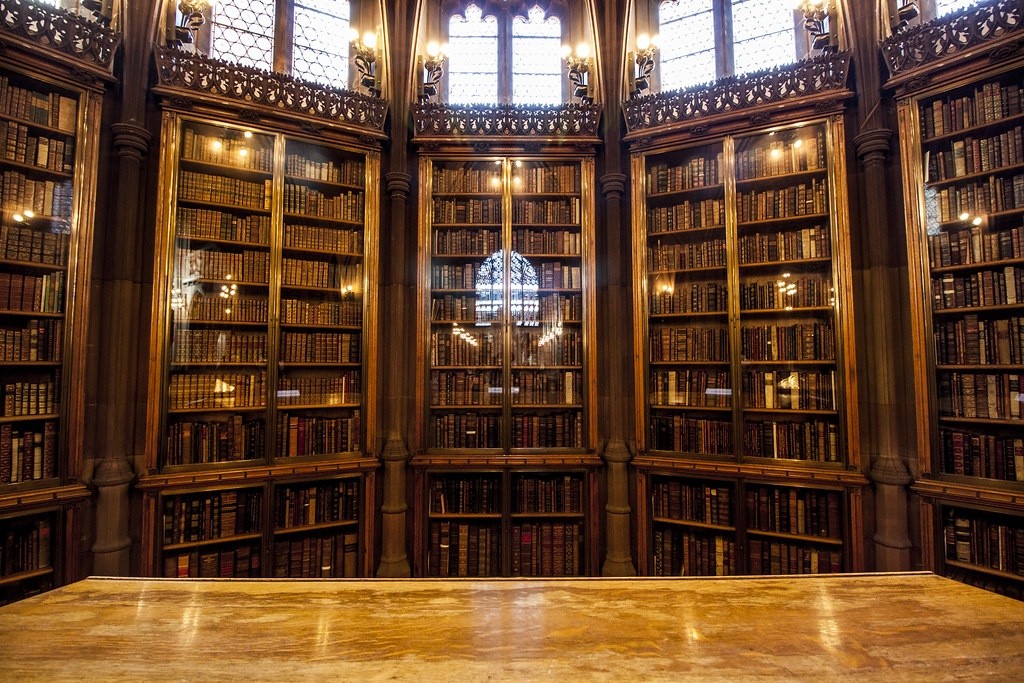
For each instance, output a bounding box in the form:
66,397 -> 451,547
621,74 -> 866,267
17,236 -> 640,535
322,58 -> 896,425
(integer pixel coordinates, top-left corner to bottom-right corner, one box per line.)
411,152 -> 599,577
0,56 -> 106,606
140,107 -> 383,581
906,34 -> 1024,602
628,110 -> 865,579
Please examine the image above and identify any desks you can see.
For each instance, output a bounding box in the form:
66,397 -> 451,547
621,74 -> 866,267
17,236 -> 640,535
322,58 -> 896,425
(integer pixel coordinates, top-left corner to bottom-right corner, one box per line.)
1,571 -> 1024,683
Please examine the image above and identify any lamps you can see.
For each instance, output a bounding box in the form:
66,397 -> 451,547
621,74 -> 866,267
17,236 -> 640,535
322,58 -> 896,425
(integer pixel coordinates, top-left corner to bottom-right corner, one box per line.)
780,0 -> 830,48
174,0 -> 217,40
626,33 -> 666,95
556,41 -> 593,97
343,28 -> 382,94
418,40 -> 450,99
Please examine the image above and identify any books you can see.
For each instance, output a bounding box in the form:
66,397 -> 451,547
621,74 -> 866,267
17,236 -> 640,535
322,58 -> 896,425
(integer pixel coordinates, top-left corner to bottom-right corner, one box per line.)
429,158 -> 586,579
645,122 -> 848,576
154,123 -> 365,577
916,73 -> 1024,604
0,73 -> 78,604
